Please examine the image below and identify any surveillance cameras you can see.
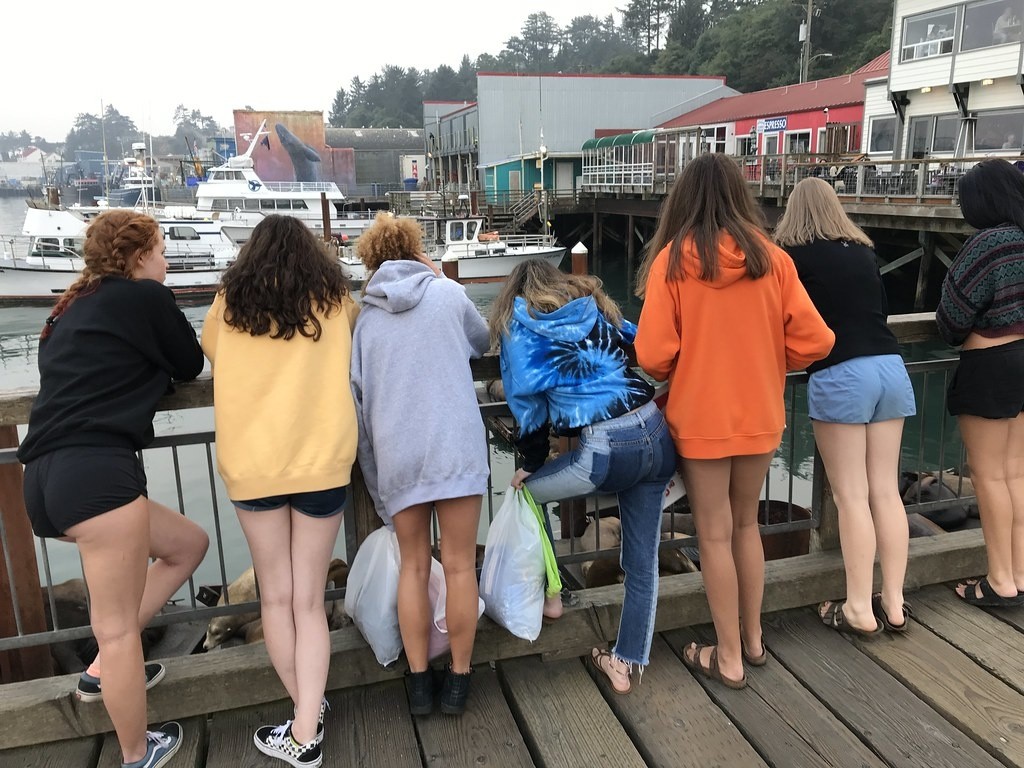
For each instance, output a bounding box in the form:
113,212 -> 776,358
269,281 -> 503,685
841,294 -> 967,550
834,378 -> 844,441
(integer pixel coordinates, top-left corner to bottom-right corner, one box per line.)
823,107 -> 829,113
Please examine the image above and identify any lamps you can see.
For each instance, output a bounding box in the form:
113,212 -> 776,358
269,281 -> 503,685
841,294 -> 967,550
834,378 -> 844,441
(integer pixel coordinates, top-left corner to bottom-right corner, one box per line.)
921,87 -> 932,94
750,126 -> 756,134
980,79 -> 994,86
824,108 -> 831,124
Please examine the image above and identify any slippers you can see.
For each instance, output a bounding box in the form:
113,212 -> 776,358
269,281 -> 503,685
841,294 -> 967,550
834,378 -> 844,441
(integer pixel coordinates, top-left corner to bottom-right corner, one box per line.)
954,577 -> 1024,607
738,617 -> 767,666
964,574 -> 1024,594
683,641 -> 747,689
818,599 -> 885,636
872,591 -> 912,632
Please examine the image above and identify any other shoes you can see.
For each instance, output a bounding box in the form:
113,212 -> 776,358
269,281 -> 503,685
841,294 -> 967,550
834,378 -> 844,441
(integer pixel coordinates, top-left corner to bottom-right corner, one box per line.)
438,664 -> 473,715
403,666 -> 437,715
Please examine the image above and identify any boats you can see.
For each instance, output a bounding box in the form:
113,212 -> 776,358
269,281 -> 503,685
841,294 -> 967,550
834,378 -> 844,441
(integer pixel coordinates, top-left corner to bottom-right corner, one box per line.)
41,98 -> 163,208
340,216 -> 568,285
0,119 -> 398,305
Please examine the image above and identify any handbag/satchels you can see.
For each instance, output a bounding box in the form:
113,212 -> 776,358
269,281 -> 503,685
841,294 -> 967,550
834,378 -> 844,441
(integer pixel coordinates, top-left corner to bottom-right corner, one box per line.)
427,555 -> 485,660
522,483 -> 563,595
479,486 -> 547,644
346,525 -> 404,666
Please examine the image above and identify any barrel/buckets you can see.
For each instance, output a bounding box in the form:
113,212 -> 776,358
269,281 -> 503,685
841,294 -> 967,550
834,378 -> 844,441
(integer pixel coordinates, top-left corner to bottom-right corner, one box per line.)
403,177 -> 418,190
186,176 -> 208,185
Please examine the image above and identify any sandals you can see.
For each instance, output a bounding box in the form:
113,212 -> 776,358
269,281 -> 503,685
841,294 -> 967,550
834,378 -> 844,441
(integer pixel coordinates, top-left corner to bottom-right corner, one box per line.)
591,647 -> 633,694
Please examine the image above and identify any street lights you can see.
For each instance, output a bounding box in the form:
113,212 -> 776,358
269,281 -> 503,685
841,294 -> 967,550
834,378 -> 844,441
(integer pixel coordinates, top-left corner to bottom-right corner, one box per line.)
800,53 -> 832,84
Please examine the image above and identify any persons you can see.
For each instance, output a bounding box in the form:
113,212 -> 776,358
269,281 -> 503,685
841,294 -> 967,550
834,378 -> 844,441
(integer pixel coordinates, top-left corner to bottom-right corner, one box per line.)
813,157 -> 876,191
201,214 -> 358,768
634,152 -> 835,689
487,258 -> 674,694
935,159 -> 1024,610
348,212 -> 494,715
15,208 -> 210,768
775,176 -> 916,637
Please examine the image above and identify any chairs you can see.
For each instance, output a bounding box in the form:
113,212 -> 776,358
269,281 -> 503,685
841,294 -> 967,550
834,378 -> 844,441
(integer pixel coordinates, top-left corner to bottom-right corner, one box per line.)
764,166 -> 971,195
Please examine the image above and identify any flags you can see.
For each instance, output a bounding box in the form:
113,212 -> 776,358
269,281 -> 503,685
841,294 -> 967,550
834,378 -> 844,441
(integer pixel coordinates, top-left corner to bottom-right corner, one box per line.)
261,136 -> 270,150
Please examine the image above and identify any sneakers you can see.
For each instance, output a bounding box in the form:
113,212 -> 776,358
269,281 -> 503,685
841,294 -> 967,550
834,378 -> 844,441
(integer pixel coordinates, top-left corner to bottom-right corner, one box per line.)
294,695 -> 330,741
76,663 -> 166,702
254,720 -> 323,768
120,721 -> 183,768
